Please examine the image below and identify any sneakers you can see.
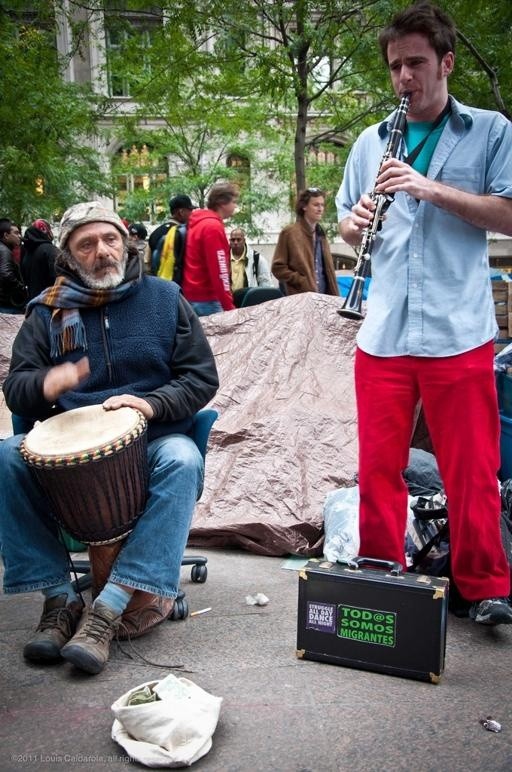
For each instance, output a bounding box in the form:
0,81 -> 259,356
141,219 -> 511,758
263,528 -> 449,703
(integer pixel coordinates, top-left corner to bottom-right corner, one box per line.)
60,600 -> 123,676
468,597 -> 512,625
23,592 -> 86,666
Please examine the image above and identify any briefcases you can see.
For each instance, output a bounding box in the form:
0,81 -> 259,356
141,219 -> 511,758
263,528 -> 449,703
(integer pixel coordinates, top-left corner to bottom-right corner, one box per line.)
294,557 -> 450,684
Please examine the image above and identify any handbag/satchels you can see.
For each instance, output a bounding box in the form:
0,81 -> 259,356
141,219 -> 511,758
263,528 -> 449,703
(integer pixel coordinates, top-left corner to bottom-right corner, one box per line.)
152,224 -> 187,285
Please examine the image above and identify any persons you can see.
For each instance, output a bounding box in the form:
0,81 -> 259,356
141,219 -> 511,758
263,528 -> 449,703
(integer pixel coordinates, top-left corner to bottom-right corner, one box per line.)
182,180 -> 241,316
271,186 -> 341,299
147,193 -> 202,286
0,215 -> 151,316
333,7 -> 512,628
2,201 -> 221,671
228,226 -> 276,291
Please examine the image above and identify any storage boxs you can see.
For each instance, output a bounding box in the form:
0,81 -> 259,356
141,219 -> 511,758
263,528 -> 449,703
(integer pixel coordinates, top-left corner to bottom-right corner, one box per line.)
498,413 -> 512,485
491,280 -> 512,354
501,373 -> 512,419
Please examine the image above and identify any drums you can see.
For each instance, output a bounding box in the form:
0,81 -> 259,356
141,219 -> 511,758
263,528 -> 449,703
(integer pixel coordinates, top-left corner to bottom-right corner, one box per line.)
20,404 -> 175,639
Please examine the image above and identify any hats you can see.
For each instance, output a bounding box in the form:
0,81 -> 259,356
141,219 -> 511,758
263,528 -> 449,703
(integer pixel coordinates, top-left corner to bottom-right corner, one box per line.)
169,194 -> 199,210
58,202 -> 129,247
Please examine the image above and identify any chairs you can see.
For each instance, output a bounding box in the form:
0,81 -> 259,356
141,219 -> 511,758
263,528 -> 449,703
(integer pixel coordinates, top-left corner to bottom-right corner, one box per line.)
12,409 -> 218,619
240,287 -> 283,307
233,287 -> 251,308
